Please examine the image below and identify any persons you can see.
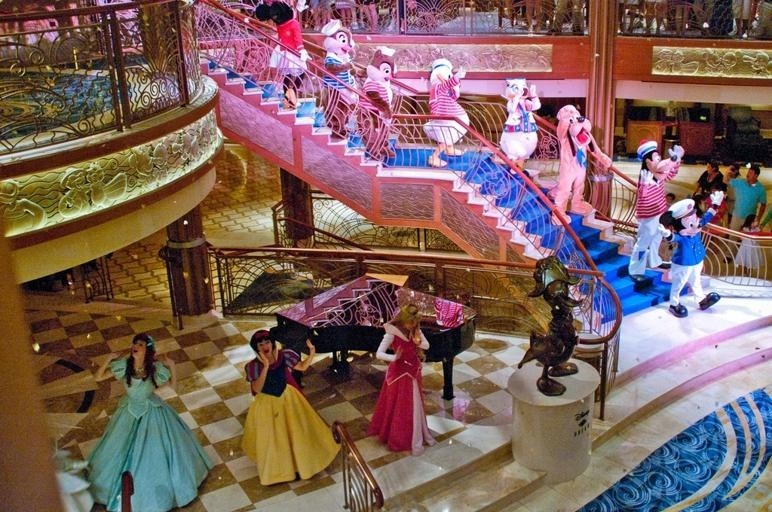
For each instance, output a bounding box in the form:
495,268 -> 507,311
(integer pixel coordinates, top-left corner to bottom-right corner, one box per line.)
366,303 -> 442,455
665,193 -> 675,208
688,158 -> 772,279
251,0 -> 771,44
85,333 -> 213,512
238,329 -> 341,486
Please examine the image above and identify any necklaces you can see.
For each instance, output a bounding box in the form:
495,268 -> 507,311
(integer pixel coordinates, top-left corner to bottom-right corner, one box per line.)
134,365 -> 143,378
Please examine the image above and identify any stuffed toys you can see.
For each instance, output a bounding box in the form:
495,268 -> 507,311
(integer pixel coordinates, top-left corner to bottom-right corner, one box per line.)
359,46 -> 397,161
551,105 -> 614,225
423,58 -> 470,166
320,19 -> 358,138
501,78 -> 541,175
628,139 -> 685,283
255,2 -> 313,109
658,191 -> 720,318
